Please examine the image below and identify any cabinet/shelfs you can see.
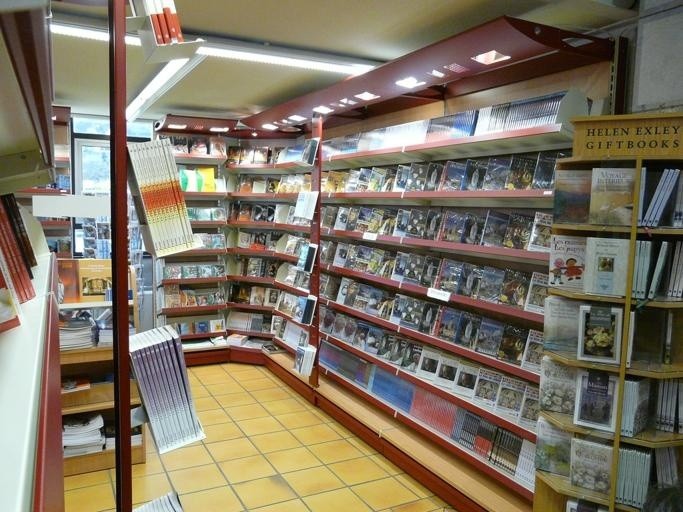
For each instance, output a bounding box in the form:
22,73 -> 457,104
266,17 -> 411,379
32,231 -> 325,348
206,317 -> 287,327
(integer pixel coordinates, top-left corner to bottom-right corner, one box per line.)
0,0 -> 65,512
153,125 -> 238,355
226,127 -> 321,368
316,120 -> 572,500
57,264 -> 146,476
533,153 -> 683,512
12,119 -> 70,260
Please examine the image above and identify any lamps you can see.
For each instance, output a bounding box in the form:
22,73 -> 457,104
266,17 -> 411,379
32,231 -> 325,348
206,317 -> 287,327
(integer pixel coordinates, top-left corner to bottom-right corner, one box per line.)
47,11 -> 390,121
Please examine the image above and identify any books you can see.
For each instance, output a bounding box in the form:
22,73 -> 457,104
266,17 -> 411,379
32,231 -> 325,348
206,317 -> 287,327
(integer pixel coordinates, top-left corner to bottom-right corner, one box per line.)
144,1 -> 183,45
0,193 -> 37,331
131,325 -> 203,451
132,491 -> 182,512
61,373 -> 142,455
164,136 -> 318,377
536,168 -> 682,507
319,151 -> 569,492
321,93 -> 568,156
43,220 -> 111,303
58,320 -> 136,350
126,138 -> 195,259
